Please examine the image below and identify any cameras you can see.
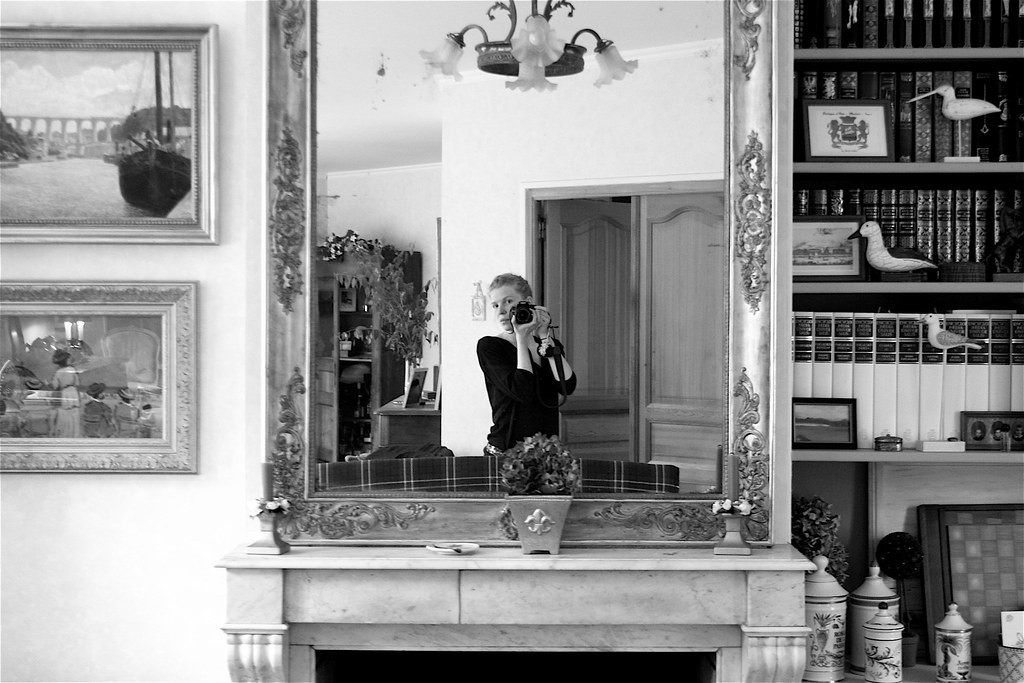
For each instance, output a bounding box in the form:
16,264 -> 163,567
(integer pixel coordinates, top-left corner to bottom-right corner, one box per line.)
514,301 -> 537,324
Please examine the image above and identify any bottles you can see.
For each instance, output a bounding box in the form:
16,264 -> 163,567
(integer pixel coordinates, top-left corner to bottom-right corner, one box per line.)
880,567 -> 897,594
863,602 -> 904,683
848,559 -> 900,675
338,383 -> 373,462
804,556 -> 849,681
934,603 -> 974,682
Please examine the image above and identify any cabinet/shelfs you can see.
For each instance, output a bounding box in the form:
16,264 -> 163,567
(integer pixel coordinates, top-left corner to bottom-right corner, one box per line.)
375,391 -> 441,457
322,251 -> 424,463
790,0 -> 1024,468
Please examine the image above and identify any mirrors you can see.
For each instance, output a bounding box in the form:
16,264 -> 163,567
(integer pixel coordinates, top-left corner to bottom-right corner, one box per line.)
262,0 -> 775,547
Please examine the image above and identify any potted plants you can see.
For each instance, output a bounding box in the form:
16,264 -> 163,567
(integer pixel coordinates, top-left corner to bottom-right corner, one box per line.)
498,433 -> 581,554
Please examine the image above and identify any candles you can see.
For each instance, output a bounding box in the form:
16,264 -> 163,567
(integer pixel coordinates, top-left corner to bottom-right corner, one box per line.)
726,452 -> 740,501
262,457 -> 276,503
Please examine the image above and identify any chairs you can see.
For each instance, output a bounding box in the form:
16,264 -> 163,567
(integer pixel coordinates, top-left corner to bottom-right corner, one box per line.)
916,502 -> 1024,666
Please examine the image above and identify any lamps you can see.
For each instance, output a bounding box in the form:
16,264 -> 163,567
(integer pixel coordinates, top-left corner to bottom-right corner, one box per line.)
419,0 -> 639,95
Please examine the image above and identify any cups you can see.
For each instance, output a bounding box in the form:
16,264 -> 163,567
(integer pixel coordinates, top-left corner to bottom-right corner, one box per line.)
997,645 -> 1024,683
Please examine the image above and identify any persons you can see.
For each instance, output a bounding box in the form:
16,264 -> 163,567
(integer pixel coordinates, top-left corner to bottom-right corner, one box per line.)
476,273 -> 577,457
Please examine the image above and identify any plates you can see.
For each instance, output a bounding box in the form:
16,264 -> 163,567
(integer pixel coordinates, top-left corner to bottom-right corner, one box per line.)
426,543 -> 479,555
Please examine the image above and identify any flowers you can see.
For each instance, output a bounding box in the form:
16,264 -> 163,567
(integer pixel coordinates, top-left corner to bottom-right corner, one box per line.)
710,498 -> 751,519
250,495 -> 292,520
314,230 -> 381,265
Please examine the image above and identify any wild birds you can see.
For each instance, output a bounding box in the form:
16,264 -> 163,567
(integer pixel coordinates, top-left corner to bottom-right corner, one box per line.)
917,313 -> 985,351
906,85 -> 1000,121
846,220 -> 939,275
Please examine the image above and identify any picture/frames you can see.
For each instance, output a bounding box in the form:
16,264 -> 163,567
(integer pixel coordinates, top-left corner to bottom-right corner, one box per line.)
340,288 -> 356,311
800,99 -> 896,162
792,397 -> 857,449
0,281 -> 198,475
0,23 -> 219,245
792,214 -> 869,283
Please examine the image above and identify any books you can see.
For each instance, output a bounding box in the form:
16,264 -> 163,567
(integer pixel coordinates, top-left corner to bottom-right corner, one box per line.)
796,188 -> 1024,283
793,311 -> 1024,451
794,0 -> 1024,49
800,69 -> 1024,163
